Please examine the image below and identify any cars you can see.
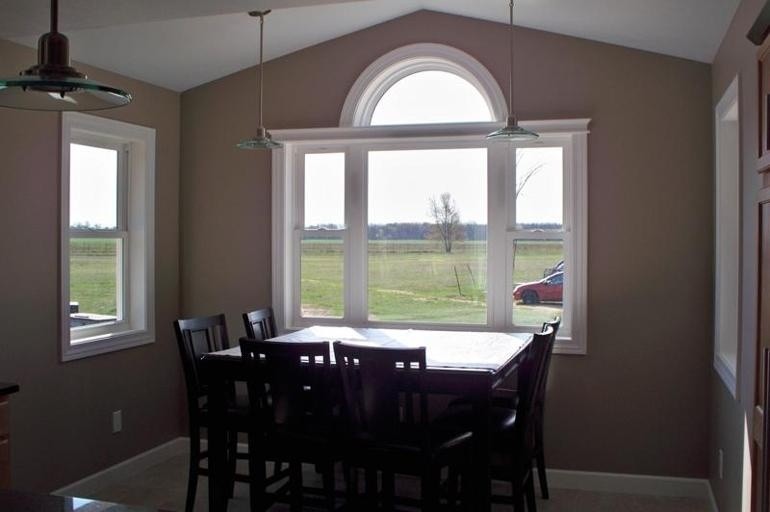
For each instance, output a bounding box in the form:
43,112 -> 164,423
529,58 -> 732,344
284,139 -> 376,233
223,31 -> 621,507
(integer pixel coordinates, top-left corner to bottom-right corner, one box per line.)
513,260 -> 563,304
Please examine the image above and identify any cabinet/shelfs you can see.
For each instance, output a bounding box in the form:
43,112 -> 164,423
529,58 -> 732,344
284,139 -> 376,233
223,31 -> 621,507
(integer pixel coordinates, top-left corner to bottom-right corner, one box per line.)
0,381 -> 20,489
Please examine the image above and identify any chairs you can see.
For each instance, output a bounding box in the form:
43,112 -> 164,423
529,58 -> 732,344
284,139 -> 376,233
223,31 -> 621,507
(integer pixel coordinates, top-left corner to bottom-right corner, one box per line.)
433,327 -> 555,511
452,316 -> 561,498
174,313 -> 302,511
239,336 -> 344,511
332,339 -> 476,511
241,308 -> 311,415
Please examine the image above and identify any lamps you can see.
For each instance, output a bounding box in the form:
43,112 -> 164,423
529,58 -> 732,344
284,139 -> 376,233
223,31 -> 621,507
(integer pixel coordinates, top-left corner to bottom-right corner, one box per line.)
0,1 -> 134,112
235,10 -> 283,153
481,0 -> 540,142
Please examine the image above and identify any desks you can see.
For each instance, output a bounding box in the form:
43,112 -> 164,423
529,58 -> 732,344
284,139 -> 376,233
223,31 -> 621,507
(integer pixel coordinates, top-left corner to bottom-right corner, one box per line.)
265,325 -> 538,399
